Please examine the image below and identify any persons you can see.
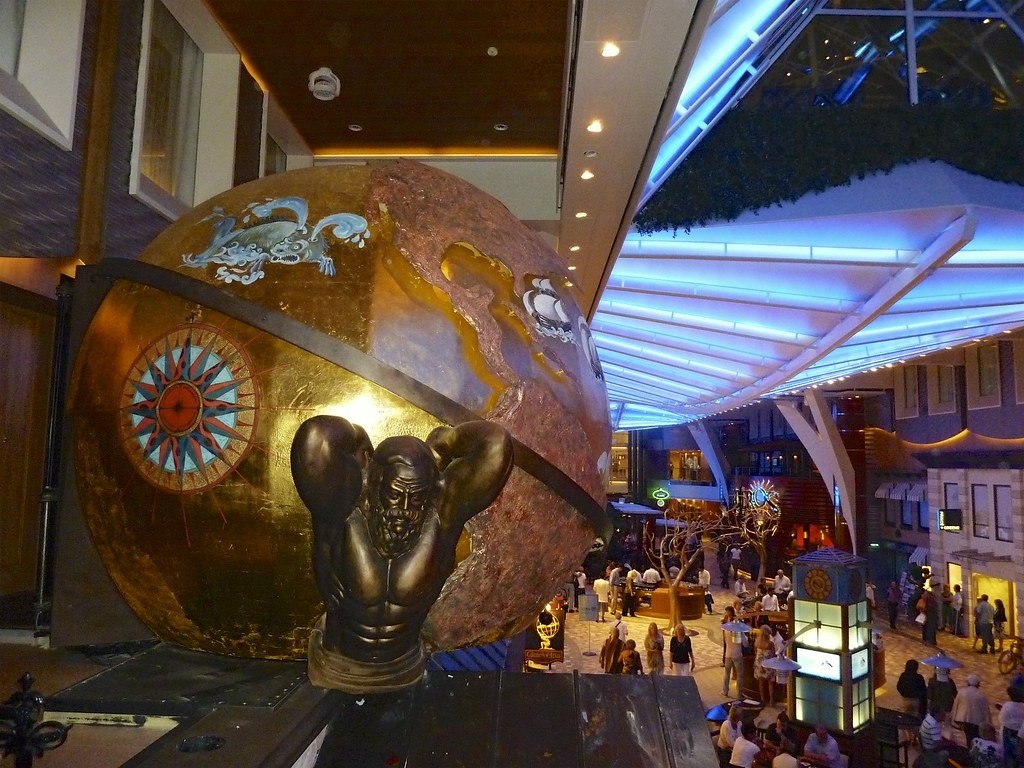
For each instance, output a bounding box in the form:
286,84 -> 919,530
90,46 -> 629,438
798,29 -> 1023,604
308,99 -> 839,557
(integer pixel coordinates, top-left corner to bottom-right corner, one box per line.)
599,612 -> 644,675
721,570 -> 791,706
972,594 -> 1008,654
885,581 -> 903,631
564,566 -> 587,613
685,532 -> 698,557
644,622 -> 664,674
289,415 -> 514,664
717,705 -> 845,768
593,561 -> 714,617
670,623 -> 695,676
896,659 -> 1024,768
865,579 -> 877,623
916,583 -> 968,645
719,543 -> 742,589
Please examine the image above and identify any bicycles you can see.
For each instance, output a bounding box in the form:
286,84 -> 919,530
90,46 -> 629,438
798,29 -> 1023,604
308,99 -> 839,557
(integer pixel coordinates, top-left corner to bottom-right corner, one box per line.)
998,643 -> 1024,674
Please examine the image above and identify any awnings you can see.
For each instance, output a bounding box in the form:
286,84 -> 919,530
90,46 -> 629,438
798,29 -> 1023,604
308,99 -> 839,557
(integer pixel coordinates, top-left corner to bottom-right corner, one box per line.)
640,518 -> 689,528
875,483 -> 928,502
908,546 -> 930,567
609,502 -> 664,514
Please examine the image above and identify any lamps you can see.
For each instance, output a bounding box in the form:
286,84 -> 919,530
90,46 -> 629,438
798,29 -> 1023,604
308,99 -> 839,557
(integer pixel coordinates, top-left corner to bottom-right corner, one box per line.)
308,66 -> 342,102
721,612 -> 789,644
761,620 -> 822,684
